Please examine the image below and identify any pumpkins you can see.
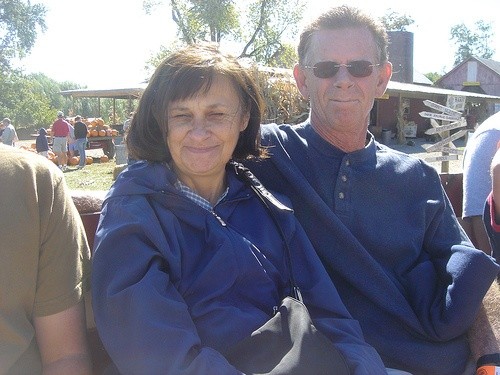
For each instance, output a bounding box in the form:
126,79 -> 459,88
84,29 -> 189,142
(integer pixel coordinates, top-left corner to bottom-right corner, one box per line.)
45,116 -> 119,137
20,143 -> 108,165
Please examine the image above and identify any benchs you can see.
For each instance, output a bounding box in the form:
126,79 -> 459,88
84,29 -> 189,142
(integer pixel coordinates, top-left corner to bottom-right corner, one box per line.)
75,173 -> 478,375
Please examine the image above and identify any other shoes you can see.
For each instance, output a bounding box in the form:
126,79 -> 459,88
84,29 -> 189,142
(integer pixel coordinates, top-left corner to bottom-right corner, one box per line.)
58,166 -> 63,171
75,164 -> 85,169
63,166 -> 68,173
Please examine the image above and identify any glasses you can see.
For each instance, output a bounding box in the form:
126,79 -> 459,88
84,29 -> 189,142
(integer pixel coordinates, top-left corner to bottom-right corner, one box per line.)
304,59 -> 381,79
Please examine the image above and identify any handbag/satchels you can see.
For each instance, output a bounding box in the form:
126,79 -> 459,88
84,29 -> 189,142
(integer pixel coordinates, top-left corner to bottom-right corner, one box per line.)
219,295 -> 350,374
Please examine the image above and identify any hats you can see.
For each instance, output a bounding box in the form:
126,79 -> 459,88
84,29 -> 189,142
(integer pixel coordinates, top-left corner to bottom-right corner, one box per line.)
57,111 -> 64,117
0,118 -> 11,123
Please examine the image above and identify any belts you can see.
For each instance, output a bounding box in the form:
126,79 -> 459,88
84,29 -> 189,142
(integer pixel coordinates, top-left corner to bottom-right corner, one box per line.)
55,136 -> 66,137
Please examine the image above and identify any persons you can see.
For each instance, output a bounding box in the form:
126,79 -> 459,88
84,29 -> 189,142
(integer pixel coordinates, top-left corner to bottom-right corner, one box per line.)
66,115 -> 88,166
0,140 -> 96,375
123,112 -> 134,131
0,118 -> 15,145
36,128 -> 50,158
238,7 -> 500,375
90,43 -> 387,375
461,110 -> 500,264
51,111 -> 69,167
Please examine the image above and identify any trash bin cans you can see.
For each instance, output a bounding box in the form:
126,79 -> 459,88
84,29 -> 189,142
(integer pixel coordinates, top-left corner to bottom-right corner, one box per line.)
115,144 -> 128,165
382,129 -> 391,145
